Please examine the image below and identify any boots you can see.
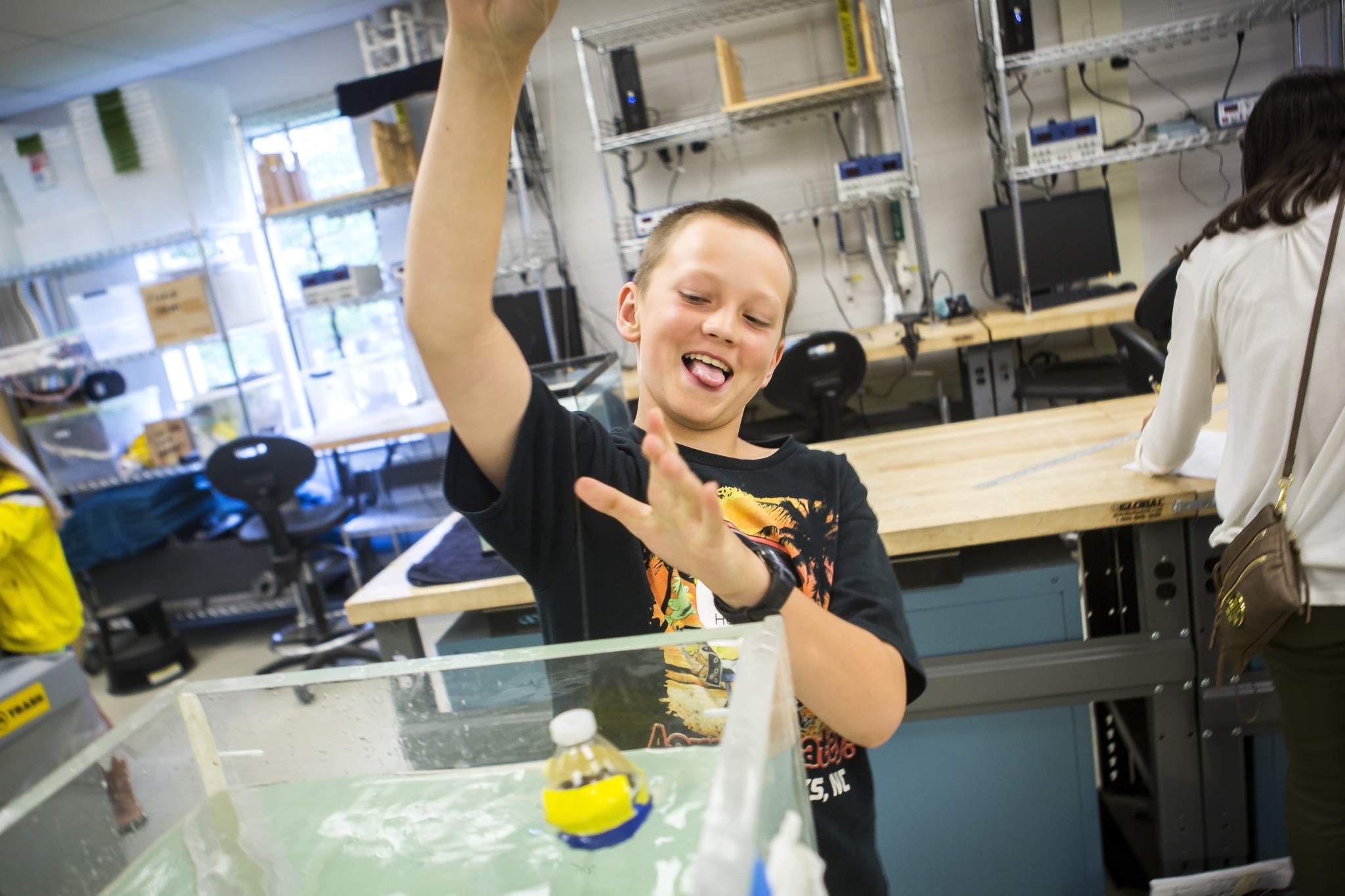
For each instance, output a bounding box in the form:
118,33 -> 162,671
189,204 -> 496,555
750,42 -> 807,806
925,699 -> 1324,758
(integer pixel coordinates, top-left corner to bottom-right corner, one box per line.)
101,757 -> 147,836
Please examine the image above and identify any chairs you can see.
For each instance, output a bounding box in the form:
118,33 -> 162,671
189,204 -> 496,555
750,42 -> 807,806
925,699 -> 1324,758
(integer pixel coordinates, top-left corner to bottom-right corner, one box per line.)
749,333 -> 873,445
203,438 -> 376,670
1104,252 -> 1188,400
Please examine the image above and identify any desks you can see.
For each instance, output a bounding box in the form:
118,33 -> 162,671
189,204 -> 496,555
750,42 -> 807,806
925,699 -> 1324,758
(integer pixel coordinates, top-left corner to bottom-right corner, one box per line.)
627,312 -> 997,421
975,268 -> 1151,414
343,382 -> 1290,895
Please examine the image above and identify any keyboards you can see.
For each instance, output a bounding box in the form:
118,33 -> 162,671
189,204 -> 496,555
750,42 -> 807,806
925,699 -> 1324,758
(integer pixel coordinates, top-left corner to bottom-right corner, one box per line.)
1007,283 -> 1117,313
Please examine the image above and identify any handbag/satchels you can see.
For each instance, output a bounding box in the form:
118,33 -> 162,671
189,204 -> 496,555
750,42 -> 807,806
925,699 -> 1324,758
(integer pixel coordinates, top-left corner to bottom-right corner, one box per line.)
1207,504 -> 1312,687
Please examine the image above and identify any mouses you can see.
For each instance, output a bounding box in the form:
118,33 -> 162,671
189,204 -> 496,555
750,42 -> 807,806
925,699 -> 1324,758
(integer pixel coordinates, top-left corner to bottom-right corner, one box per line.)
1118,282 -> 1138,292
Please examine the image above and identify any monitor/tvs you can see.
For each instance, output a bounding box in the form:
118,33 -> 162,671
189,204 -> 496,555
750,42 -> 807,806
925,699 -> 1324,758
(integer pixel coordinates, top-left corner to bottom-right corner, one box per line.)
491,285 -> 585,366
978,188 -> 1120,299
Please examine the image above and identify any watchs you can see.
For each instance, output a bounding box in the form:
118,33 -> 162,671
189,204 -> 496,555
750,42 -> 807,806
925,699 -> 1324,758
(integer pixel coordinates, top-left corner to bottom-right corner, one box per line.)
713,543 -> 797,625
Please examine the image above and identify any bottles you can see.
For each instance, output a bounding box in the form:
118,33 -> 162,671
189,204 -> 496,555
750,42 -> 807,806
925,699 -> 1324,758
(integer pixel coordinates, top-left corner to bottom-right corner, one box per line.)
543,708 -> 658,895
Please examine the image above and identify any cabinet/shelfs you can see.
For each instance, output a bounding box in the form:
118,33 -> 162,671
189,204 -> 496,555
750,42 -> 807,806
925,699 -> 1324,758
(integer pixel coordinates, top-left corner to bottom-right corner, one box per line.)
974,0 -> 1345,317
572,0 -> 938,324
226,61 -> 569,636
0,227 -> 350,630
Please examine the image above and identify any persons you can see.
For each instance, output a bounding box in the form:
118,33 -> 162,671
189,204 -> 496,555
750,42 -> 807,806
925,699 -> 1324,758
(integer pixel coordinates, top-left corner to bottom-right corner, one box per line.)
1132,65 -> 1344,896
1,434 -> 149,836
403,1 -> 929,895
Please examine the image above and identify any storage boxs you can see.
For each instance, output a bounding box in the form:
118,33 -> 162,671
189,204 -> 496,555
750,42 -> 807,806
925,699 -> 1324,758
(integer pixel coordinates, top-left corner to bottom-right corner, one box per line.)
19,371 -> 287,488
528,352 -> 637,435
0,76 -> 245,273
300,264 -> 383,308
66,272 -> 158,354
1,652 -> 126,896
1,614 -> 828,895
140,270 -> 270,347
